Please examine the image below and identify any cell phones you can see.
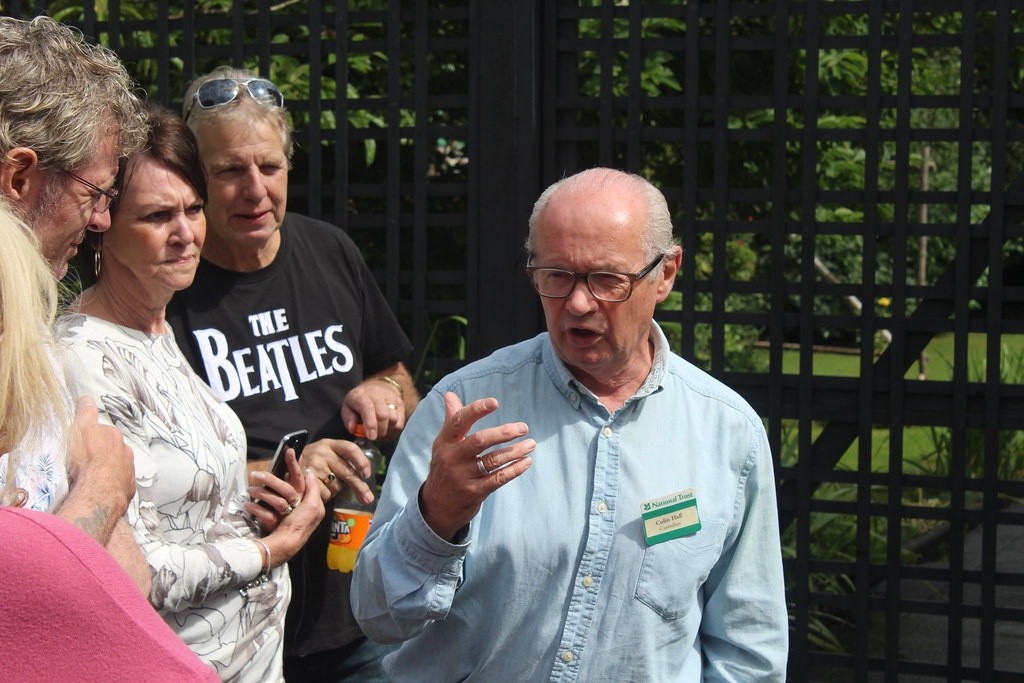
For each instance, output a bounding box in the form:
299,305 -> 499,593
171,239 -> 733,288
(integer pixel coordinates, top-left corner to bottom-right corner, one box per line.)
250,429 -> 309,519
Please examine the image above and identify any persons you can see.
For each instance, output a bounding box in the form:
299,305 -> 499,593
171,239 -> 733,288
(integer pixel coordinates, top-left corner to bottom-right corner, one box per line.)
349,166 -> 791,682
0,39 -> 424,682
1,204 -> 226,683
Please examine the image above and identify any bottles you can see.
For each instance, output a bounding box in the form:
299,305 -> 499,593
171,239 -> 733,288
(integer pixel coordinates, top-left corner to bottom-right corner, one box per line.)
326,423 -> 383,573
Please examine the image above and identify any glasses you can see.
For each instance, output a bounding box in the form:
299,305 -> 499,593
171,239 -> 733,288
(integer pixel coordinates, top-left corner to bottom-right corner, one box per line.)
525,253 -> 666,303
184,77 -> 285,122
59,167 -> 120,213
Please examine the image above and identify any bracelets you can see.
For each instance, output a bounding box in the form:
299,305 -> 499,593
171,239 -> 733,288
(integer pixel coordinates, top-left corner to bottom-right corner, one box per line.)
245,537 -> 272,591
376,376 -> 404,400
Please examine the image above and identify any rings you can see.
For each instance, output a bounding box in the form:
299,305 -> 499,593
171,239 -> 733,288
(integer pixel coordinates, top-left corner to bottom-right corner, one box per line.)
325,473 -> 335,484
279,496 -> 301,518
387,403 -> 398,410
476,457 -> 489,475
